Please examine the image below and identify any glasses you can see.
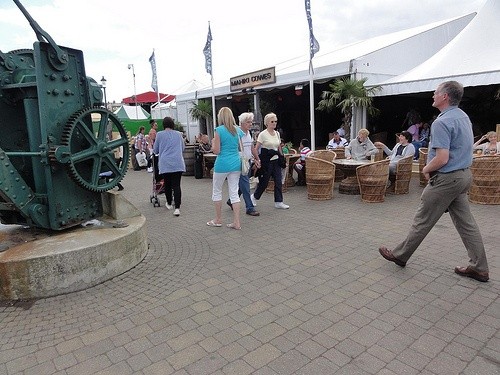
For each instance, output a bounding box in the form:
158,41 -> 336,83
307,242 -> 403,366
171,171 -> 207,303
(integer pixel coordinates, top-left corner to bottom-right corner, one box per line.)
434,90 -> 450,96
269,121 -> 277,123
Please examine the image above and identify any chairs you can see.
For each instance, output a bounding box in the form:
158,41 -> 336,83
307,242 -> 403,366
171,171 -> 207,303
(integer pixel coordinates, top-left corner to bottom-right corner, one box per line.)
303,154 -> 335,202
389,155 -> 415,195
264,154 -> 293,193
308,148 -> 339,159
419,147 -> 430,186
371,145 -> 385,160
468,154 -> 500,205
332,147 -> 345,181
289,148 -> 298,155
357,158 -> 391,203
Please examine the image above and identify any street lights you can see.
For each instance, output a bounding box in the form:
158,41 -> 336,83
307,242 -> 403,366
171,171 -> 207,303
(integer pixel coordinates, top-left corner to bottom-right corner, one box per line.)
100,75 -> 107,110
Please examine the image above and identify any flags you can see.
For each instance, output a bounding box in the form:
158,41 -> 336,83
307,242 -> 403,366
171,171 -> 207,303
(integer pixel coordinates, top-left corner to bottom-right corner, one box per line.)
304,0 -> 319,75
148,51 -> 159,93
202,24 -> 214,74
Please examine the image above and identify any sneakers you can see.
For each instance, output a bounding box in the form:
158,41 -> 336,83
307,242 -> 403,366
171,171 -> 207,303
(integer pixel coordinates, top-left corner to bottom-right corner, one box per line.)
250,194 -> 256,207
275,202 -> 289,209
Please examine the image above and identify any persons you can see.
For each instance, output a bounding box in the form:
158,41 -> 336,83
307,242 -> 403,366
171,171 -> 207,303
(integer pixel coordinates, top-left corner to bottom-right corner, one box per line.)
130,121 -> 191,173
197,134 -> 212,154
152,116 -> 186,217
373,132 -> 416,188
280,140 -> 288,154
327,124 -> 349,149
250,112 -> 290,210
473,131 -> 500,156
292,139 -> 311,182
226,111 -> 262,217
407,115 -> 436,160
378,81 -> 491,283
206,107 -> 243,231
345,128 -> 378,175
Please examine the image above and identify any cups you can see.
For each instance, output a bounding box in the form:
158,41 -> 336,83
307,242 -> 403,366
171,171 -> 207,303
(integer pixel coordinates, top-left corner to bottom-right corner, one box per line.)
371,155 -> 375,162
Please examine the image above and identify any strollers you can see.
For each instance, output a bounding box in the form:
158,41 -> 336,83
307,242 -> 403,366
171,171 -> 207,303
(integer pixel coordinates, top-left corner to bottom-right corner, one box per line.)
146,153 -> 175,207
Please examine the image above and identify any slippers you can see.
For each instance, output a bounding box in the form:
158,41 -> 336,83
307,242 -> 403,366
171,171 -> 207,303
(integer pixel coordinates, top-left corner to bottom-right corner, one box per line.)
227,224 -> 241,230
206,220 -> 222,227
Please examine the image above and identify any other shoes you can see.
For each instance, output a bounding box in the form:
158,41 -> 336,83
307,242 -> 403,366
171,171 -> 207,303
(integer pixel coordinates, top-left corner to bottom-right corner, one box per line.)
173,208 -> 180,215
150,167 -> 154,172
413,157 -> 419,160
147,168 -> 152,172
166,202 -> 173,210
227,199 -> 233,211
246,211 -> 260,216
386,183 -> 395,192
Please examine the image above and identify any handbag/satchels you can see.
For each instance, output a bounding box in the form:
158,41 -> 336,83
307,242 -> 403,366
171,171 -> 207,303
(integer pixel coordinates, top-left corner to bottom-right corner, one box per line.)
135,152 -> 148,167
235,125 -> 249,176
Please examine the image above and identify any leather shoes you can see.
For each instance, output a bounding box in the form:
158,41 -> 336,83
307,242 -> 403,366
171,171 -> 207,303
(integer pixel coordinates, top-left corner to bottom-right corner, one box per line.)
455,265 -> 489,282
379,247 -> 406,268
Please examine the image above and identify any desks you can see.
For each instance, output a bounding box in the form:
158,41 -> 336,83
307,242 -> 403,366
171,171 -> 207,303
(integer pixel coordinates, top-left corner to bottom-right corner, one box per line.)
334,157 -> 381,194
203,152 -> 217,178
287,153 -> 301,187
